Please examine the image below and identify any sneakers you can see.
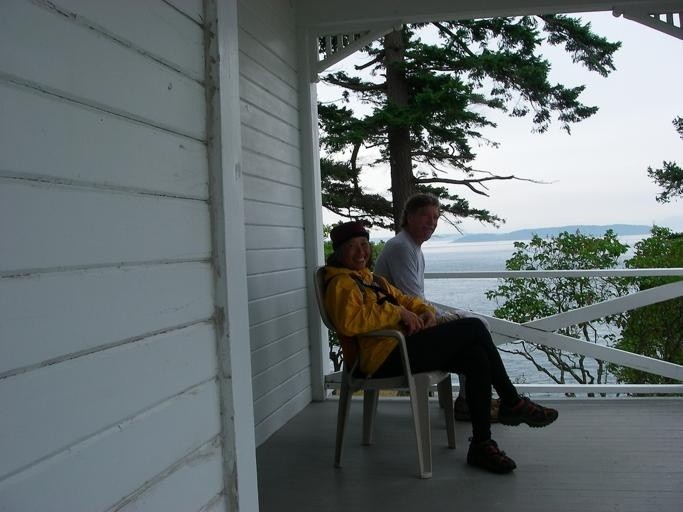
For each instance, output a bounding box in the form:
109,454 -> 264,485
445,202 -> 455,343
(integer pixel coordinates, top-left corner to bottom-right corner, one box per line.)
497,396 -> 559,429
466,439 -> 517,474
453,397 -> 499,423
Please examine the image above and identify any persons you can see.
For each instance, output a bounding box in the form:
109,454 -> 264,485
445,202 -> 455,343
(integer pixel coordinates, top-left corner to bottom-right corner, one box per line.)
315,220 -> 558,473
372,192 -> 502,426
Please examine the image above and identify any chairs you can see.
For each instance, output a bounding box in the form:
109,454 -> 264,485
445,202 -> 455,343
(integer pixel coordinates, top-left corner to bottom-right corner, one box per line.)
314,265 -> 457,479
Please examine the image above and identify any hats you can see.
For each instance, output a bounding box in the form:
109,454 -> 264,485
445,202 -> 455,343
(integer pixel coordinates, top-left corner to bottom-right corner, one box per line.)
330,221 -> 370,250
